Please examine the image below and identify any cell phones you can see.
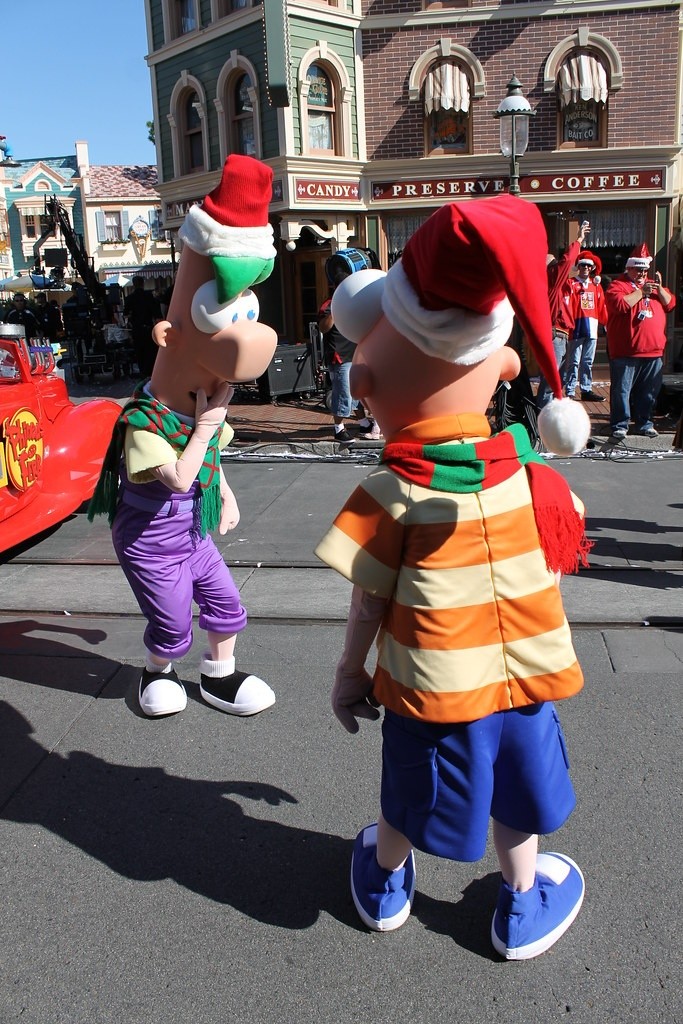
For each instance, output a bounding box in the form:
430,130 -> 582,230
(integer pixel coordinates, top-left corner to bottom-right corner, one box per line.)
654,273 -> 658,283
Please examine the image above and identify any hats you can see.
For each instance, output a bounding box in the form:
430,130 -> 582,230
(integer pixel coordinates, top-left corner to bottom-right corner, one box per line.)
33,292 -> 45,299
572,249 -> 603,285
625,241 -> 653,270
177,153 -> 280,263
377,190 -> 589,459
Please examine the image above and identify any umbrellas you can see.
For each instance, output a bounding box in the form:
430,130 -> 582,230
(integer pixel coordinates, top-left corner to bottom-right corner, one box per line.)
0,273 -> 72,293
101,271 -> 134,308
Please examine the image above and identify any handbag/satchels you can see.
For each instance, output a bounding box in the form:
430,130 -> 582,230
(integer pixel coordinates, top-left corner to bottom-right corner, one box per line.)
612,429 -> 629,439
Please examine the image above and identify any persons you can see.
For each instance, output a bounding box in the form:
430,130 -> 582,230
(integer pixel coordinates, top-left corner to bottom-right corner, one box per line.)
317,270 -> 375,441
604,241 -> 676,438
564,249 -> 606,401
86,153 -> 277,716
6,293 -> 45,346
537,224 -> 591,408
34,292 -> 57,338
66,276 -> 174,382
314,192 -> 595,962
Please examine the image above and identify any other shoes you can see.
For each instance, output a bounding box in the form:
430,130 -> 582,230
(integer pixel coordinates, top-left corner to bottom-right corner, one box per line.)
199,655 -> 276,717
332,426 -> 355,444
137,659 -> 187,718
581,389 -> 607,402
490,852 -> 585,961
350,822 -> 417,933
358,422 -> 375,439
636,427 -> 658,437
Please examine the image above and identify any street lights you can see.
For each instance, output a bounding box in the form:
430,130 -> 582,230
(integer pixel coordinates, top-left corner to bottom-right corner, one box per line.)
491,69 -> 539,197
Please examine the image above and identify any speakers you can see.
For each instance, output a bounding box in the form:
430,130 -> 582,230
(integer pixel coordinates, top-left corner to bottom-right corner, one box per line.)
256,344 -> 317,396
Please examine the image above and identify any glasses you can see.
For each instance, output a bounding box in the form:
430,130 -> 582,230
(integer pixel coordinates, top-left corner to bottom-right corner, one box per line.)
578,263 -> 591,267
14,300 -> 23,302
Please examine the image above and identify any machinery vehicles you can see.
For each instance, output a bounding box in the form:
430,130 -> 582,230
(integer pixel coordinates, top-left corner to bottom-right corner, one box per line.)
31,192 -> 128,371
